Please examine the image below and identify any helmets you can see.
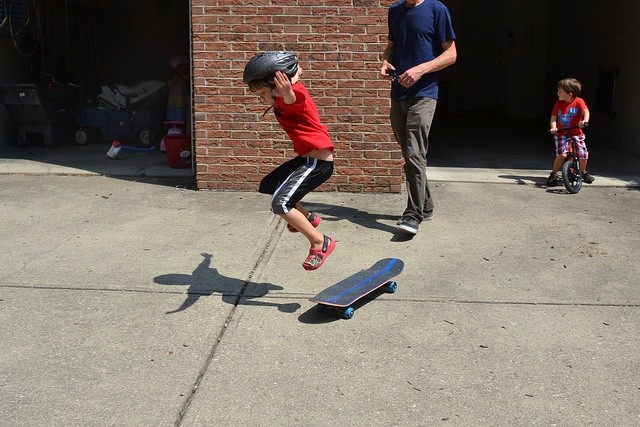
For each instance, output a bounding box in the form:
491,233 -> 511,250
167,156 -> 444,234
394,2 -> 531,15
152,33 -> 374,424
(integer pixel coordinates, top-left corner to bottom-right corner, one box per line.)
243,51 -> 299,84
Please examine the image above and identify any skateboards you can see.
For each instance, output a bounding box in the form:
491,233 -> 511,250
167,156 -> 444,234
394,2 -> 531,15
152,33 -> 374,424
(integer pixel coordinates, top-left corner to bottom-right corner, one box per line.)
308,258 -> 404,317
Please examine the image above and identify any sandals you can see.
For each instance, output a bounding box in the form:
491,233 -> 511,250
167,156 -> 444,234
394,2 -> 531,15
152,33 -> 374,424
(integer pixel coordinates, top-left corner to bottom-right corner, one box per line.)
287,211 -> 320,233
302,235 -> 335,270
547,172 -> 558,187
582,171 -> 592,183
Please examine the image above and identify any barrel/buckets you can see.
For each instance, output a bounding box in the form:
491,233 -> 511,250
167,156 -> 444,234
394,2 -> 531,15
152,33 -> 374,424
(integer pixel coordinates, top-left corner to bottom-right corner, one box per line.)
165,134 -> 192,169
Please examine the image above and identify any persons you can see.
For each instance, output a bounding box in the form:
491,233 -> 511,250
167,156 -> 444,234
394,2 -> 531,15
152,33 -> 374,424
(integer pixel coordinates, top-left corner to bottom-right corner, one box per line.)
545,77 -> 595,187
381,0 -> 459,237
242,50 -> 336,270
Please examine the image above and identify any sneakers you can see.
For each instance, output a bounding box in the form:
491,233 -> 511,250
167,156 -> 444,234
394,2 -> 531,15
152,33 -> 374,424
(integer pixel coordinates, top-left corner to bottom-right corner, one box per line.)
423,215 -> 434,219
394,215 -> 419,234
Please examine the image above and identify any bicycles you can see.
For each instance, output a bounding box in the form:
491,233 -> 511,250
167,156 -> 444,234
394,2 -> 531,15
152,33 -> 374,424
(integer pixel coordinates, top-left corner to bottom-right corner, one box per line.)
548,122 -> 592,193
